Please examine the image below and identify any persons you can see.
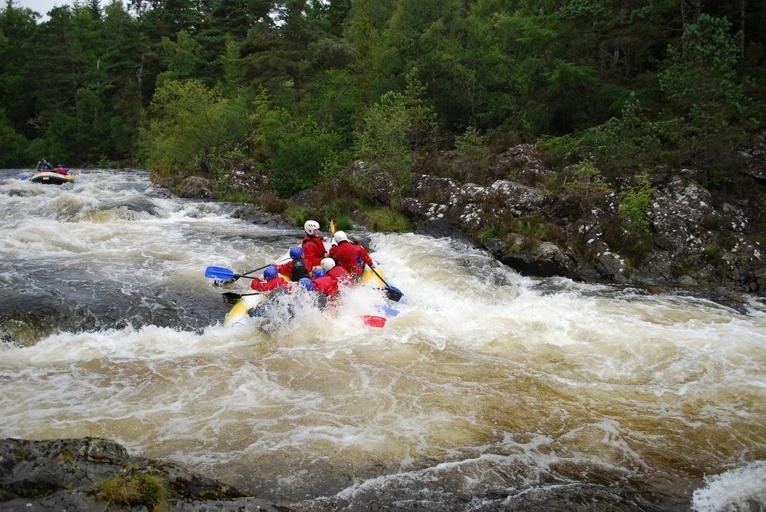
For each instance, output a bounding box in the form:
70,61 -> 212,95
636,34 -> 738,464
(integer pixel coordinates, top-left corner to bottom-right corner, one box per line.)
32,159 -> 68,175
251,220 -> 372,318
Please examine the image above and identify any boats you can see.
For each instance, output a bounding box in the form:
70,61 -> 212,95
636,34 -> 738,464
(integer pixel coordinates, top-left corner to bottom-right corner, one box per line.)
222,251 -> 388,333
29,170 -> 75,188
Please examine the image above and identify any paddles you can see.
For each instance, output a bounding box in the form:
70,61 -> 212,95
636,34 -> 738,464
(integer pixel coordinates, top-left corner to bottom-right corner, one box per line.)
350,266 -> 404,327
206,266 -> 273,299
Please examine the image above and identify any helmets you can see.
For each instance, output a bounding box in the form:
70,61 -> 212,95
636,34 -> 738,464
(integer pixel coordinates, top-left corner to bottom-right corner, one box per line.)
263,266 -> 280,280
334,230 -> 351,244
289,246 -> 304,259
311,256 -> 337,278
298,277 -> 315,293
304,219 -> 320,235
